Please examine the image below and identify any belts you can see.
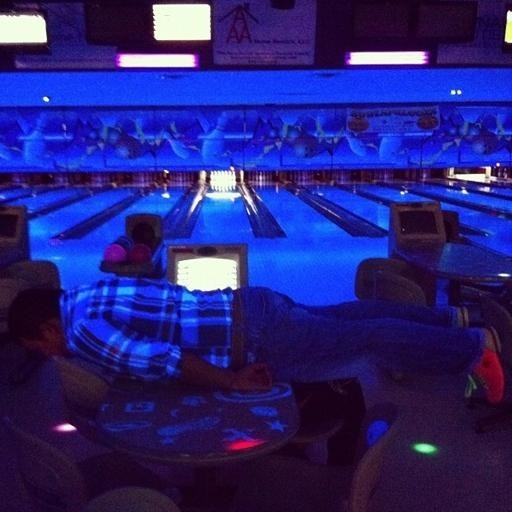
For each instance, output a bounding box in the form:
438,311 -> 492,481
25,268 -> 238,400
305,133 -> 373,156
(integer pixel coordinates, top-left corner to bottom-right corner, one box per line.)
233,290 -> 242,362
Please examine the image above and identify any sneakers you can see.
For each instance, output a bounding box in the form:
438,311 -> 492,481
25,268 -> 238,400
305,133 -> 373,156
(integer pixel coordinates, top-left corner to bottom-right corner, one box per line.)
457,306 -> 506,406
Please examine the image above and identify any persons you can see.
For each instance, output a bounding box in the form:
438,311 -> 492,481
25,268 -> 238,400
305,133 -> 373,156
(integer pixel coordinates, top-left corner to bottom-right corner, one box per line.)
9,275 -> 506,408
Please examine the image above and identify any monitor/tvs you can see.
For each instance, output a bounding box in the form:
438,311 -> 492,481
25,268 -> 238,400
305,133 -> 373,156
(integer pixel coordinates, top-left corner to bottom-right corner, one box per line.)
0,3 -> 49,44
153,5 -> 212,43
167,242 -> 248,292
0,205 -> 25,244
390,200 -> 446,240
